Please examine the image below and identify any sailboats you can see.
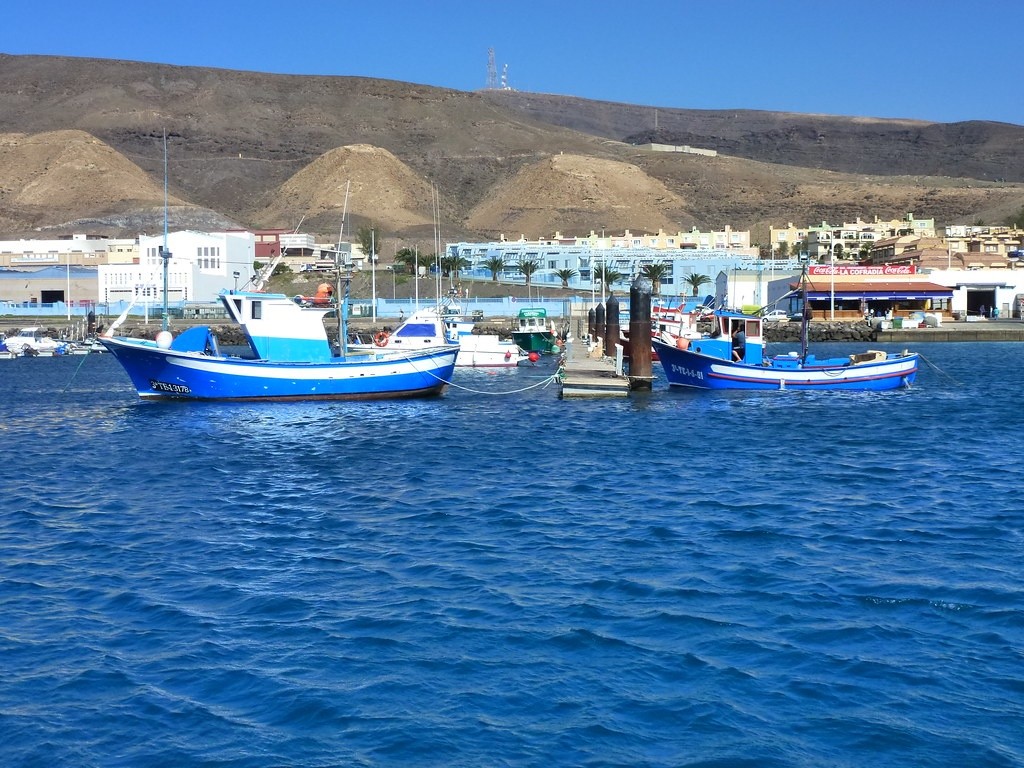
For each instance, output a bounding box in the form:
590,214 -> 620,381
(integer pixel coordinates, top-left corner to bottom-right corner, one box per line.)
331,180 -> 540,368
96,129 -> 462,403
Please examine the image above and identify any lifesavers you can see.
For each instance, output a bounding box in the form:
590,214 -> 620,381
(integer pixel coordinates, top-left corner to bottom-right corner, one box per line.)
374,331 -> 389,348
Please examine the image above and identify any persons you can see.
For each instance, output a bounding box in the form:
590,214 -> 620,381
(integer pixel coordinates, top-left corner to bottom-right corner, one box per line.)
868,306 -> 890,316
980,305 -> 985,316
989,305 -> 993,317
378,326 -> 393,343
326,290 -> 337,317
994,307 -> 1000,320
731,324 -> 746,362
399,309 -> 404,322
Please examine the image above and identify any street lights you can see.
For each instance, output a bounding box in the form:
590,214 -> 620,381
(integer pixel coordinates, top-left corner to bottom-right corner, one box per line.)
371,227 -> 375,324
827,228 -> 834,321
413,243 -> 418,308
601,226 -> 607,307
387,266 -> 396,299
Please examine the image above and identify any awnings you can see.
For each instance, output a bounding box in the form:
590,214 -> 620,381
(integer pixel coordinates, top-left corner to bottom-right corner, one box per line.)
782,291 -> 803,298
803,290 -> 955,301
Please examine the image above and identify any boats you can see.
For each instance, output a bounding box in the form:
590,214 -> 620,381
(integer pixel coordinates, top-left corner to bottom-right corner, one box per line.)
619,302 -> 702,362
0,251 -> 111,360
651,248 -> 921,392
509,308 -> 557,354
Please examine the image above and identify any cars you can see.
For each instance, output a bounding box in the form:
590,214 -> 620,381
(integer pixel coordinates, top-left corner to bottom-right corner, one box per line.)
761,309 -> 787,322
787,311 -> 803,322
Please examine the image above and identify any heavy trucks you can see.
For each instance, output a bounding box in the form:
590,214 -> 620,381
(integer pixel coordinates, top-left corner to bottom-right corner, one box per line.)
299,260 -> 336,273
